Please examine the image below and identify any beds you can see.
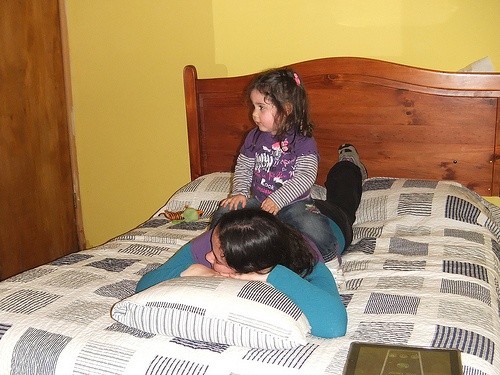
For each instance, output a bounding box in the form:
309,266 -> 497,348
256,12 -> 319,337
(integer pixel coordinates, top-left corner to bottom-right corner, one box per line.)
0,170 -> 499,375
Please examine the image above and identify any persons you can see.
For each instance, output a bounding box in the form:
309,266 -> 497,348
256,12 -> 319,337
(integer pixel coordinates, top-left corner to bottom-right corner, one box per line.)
208,68 -> 349,293
135,141 -> 369,338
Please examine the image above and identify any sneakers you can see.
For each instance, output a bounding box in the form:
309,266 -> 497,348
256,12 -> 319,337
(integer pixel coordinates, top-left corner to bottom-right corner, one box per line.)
338,143 -> 368,181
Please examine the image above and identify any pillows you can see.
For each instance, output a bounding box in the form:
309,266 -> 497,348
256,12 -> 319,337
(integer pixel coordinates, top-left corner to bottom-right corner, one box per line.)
109,273 -> 312,351
